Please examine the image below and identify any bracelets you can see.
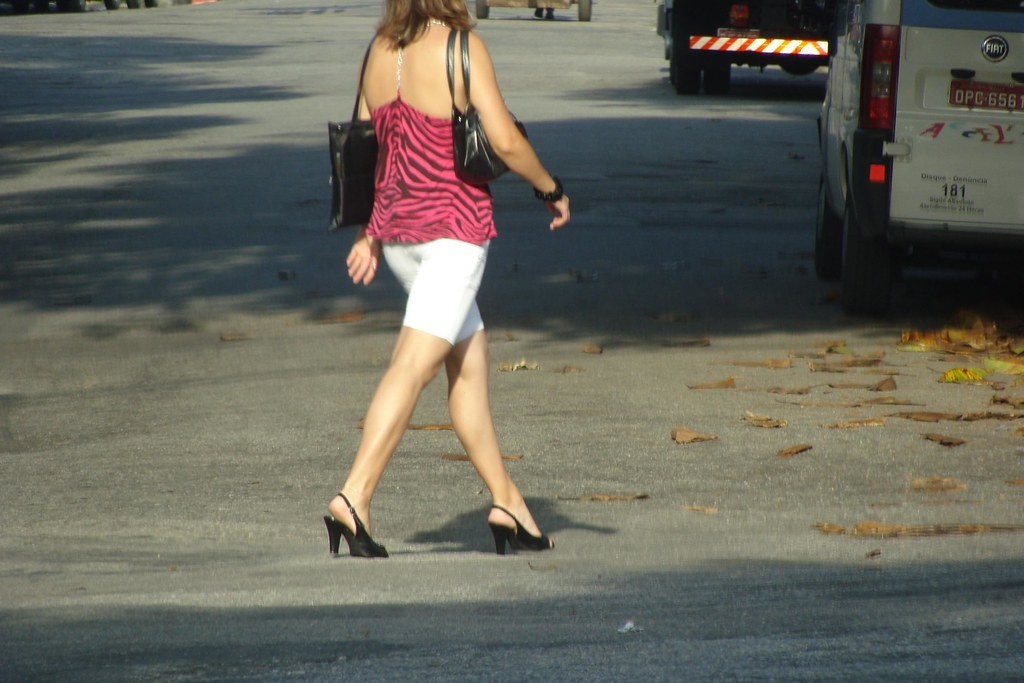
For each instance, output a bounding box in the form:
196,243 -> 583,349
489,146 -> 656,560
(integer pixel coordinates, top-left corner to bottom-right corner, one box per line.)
534,176 -> 565,203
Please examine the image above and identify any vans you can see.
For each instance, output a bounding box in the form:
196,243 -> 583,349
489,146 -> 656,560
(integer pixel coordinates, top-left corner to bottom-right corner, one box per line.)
815,0 -> 1024,296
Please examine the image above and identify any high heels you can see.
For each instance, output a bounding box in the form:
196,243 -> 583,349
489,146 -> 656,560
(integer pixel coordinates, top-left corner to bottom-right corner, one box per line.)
323,493 -> 389,558
488,505 -> 556,555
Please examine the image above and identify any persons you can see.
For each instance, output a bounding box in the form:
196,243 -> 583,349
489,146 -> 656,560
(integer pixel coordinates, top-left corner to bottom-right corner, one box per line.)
324,1 -> 571,560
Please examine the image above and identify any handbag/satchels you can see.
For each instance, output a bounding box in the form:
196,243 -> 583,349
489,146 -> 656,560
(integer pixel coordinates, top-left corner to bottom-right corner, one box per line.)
328,24 -> 379,232
445,27 -> 532,187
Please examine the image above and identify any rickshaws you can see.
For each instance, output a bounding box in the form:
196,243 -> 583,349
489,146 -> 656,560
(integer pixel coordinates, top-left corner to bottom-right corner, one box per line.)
474,0 -> 593,24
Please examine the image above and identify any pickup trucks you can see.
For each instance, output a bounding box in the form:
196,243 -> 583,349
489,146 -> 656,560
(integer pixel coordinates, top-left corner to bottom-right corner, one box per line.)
652,0 -> 829,95
